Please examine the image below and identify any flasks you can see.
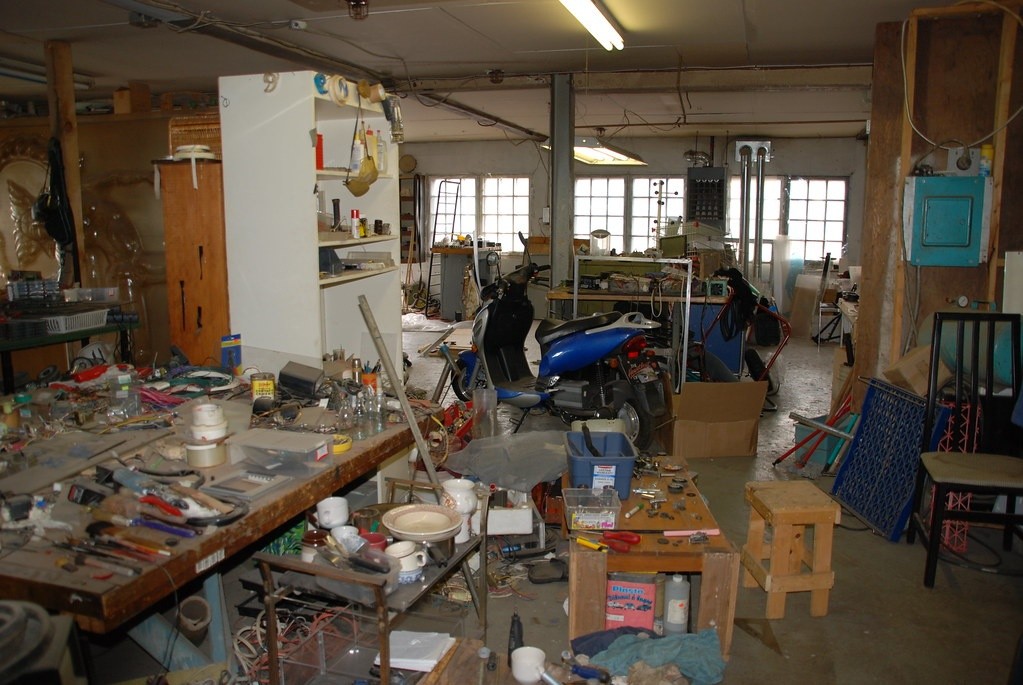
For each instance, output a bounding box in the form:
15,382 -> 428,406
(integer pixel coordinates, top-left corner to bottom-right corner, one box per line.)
353,359 -> 362,384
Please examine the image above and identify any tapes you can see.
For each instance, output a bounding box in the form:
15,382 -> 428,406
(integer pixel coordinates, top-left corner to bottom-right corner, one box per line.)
184,404 -> 229,466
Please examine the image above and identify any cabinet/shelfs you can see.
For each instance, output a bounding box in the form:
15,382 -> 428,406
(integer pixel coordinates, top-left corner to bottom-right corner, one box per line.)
265,483 -> 493,685
150,70 -> 404,387
398,173 -> 425,263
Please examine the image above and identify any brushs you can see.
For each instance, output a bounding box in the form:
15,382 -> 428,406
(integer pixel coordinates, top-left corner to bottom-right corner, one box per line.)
53,483 -> 201,582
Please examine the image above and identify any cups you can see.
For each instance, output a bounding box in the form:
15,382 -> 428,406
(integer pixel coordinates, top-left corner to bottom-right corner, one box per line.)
317,497 -> 349,529
471,389 -> 498,440
331,526 -> 359,538
360,532 -> 388,551
385,541 -> 427,571
362,374 -> 376,392
511,646 -> 546,685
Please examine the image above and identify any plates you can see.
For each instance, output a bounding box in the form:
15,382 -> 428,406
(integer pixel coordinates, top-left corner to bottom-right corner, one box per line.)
382,504 -> 464,542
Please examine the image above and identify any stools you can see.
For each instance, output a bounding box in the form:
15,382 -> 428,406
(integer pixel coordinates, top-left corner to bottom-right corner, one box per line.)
740,478 -> 844,619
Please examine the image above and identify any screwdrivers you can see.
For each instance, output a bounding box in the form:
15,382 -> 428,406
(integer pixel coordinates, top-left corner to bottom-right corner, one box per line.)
578,528 -> 642,544
551,662 -> 609,682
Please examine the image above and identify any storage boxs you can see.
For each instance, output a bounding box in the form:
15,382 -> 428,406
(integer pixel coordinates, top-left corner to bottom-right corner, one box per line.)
561,428 -> 638,501
561,487 -> 623,533
470,492 -> 535,534
62,287 -> 82,302
223,425 -> 339,474
792,414 -> 853,465
605,571 -> 657,631
652,367 -> 769,456
665,221 -> 725,251
881,343 -> 953,398
80,287 -> 120,302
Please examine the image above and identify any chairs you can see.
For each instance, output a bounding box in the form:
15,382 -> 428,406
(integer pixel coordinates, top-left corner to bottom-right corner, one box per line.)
816,283 -> 845,350
904,310 -> 1023,589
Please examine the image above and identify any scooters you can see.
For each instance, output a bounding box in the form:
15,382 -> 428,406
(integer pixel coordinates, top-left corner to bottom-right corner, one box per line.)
451,230 -> 669,452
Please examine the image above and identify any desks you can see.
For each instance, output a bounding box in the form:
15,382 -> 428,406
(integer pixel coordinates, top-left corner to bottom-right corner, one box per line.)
565,453 -> 734,650
0,320 -> 142,396
837,298 -> 859,348
0,370 -> 439,685
546,282 -> 731,379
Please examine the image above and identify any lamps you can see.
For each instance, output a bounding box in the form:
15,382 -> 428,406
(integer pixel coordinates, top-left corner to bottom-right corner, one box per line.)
540,127 -> 649,167
557,0 -> 626,51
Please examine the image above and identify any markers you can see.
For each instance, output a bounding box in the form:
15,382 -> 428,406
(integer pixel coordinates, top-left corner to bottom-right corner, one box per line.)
624,503 -> 644,518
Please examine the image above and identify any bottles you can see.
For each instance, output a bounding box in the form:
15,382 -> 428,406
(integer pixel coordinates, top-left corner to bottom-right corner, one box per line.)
663,575 -> 690,637
338,392 -> 385,439
350,123 -> 386,174
300,529 -> 329,563
350,209 -> 360,235
508,610 -> 524,667
442,478 -> 478,544
374,219 -> 382,235
